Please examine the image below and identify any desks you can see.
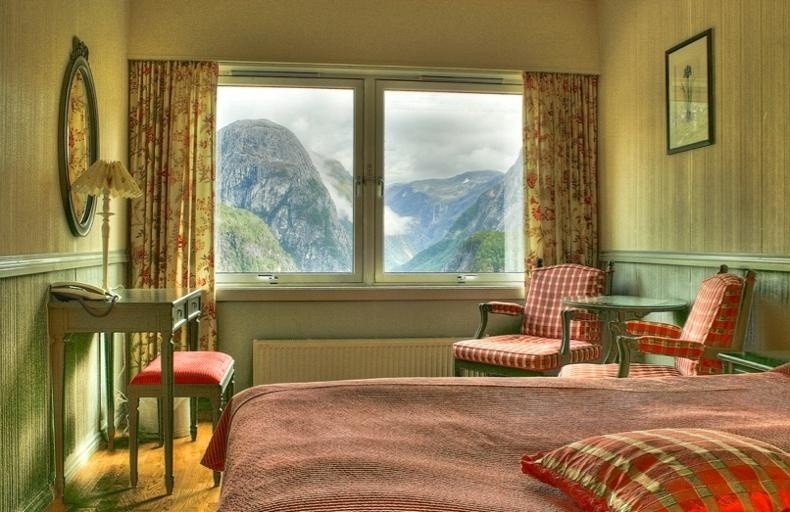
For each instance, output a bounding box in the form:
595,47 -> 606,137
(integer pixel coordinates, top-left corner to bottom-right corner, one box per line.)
48,287 -> 203,495
564,295 -> 690,362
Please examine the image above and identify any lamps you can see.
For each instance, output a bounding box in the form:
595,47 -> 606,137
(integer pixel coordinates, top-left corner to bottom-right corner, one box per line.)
73,158 -> 145,294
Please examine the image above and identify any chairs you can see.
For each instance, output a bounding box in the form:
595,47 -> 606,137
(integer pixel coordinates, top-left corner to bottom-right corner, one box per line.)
451,263 -> 615,373
562,271 -> 749,383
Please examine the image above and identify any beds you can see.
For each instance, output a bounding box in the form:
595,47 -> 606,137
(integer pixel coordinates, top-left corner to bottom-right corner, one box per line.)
222,371 -> 788,512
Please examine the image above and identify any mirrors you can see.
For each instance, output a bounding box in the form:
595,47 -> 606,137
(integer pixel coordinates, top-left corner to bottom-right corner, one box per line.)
62,44 -> 100,239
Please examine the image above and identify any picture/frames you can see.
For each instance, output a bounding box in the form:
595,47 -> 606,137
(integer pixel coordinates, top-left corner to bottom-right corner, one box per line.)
663,26 -> 716,151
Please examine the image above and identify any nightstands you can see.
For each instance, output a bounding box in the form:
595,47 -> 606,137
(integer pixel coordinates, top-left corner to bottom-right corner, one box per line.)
714,351 -> 782,375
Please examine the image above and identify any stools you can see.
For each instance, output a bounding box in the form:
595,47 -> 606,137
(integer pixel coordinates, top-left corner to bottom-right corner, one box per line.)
126,349 -> 236,484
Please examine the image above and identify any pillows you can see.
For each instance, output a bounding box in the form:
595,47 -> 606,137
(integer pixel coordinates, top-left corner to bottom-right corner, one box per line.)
524,423 -> 790,512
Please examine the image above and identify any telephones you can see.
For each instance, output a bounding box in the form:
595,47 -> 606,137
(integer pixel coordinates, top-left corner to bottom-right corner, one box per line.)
48,282 -> 121,301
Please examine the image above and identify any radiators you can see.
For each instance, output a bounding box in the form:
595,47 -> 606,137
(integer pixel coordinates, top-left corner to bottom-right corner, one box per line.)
250,335 -> 504,382
